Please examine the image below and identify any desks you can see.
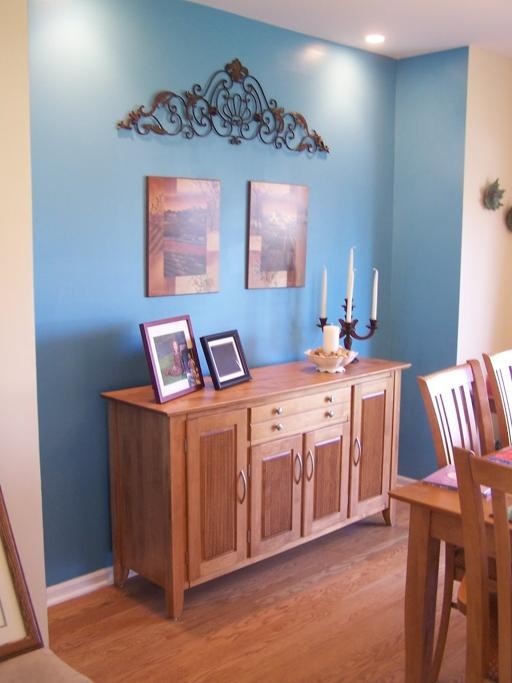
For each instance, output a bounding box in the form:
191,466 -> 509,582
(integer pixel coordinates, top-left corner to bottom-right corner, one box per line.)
379,444 -> 512,683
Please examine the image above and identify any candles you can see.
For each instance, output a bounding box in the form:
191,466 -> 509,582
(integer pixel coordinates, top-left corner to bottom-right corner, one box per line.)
319,245 -> 379,324
321,325 -> 340,353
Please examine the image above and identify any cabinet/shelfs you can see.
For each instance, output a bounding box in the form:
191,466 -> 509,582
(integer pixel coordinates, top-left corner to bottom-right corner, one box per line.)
96,358 -> 412,622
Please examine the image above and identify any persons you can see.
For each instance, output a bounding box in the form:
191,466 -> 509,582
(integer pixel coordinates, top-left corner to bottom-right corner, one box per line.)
162,340 -> 181,378
186,351 -> 198,384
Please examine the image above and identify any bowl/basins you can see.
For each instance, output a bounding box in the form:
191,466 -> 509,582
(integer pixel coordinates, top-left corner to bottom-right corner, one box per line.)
304,348 -> 360,374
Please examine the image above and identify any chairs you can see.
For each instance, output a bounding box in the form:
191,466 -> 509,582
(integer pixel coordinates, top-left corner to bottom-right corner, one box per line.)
415,343 -> 512,683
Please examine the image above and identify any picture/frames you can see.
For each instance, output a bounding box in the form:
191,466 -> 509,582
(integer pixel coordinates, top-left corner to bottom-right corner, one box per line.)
138,313 -> 253,404
